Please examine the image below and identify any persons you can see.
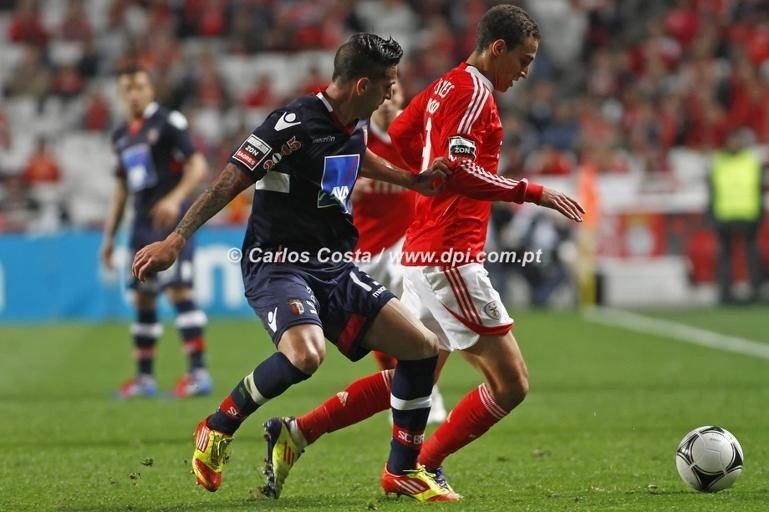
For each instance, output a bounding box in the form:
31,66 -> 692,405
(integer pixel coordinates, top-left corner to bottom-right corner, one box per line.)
131,33 -> 469,502
344,74 -> 452,425
102,64 -> 213,400
260,3 -> 585,497
0,1 -> 768,231
702,126 -> 769,304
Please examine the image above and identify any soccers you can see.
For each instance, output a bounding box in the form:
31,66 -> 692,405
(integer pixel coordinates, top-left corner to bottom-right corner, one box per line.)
675,426 -> 744,492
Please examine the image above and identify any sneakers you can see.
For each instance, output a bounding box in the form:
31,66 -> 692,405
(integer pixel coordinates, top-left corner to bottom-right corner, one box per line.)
382,458 -> 465,502
190,414 -> 233,492
173,368 -> 212,397
114,374 -> 156,399
263,416 -> 305,500
